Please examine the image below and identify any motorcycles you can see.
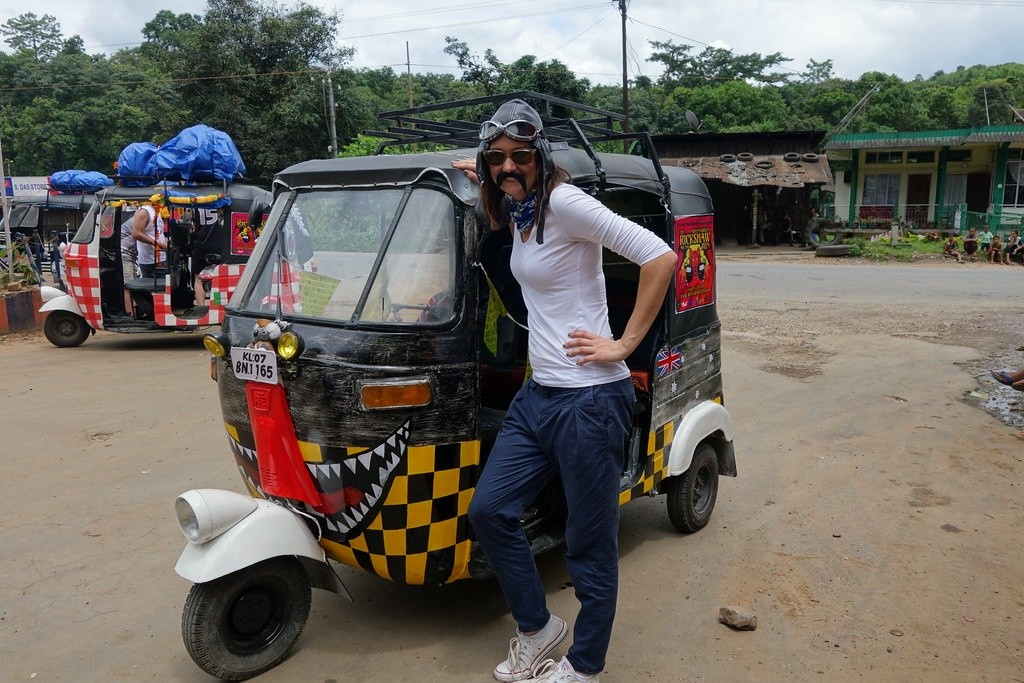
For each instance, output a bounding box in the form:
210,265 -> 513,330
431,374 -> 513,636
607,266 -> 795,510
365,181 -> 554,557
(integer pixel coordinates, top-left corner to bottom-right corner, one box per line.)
0,194 -> 97,291
36,183 -> 319,347
176,136 -> 738,683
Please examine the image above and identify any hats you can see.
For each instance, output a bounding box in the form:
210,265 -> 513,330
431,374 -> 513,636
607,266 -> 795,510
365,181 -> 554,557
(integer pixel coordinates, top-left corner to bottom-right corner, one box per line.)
473,97 -> 556,244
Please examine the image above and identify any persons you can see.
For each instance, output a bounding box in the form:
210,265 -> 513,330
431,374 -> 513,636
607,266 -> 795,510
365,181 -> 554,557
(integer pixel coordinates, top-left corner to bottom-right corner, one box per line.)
944,233 -> 966,263
962,227 -> 978,257
194,256 -> 206,307
133,203 -> 170,278
453,99 -> 677,683
1004,230 -> 1024,265
987,236 -> 1003,265
977,225 -> 994,254
121,217 -> 139,314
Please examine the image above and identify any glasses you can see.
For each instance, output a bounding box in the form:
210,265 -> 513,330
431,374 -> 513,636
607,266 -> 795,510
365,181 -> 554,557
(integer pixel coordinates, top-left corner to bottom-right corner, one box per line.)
478,119 -> 546,142
481,148 -> 537,164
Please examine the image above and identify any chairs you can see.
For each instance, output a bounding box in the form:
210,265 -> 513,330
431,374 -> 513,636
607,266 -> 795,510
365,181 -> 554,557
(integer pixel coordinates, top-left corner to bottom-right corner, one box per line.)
123,246 -> 180,320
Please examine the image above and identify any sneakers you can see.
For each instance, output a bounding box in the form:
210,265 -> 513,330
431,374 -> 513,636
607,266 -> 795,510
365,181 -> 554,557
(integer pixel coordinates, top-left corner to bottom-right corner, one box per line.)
492,613 -> 568,683
512,655 -> 601,683
